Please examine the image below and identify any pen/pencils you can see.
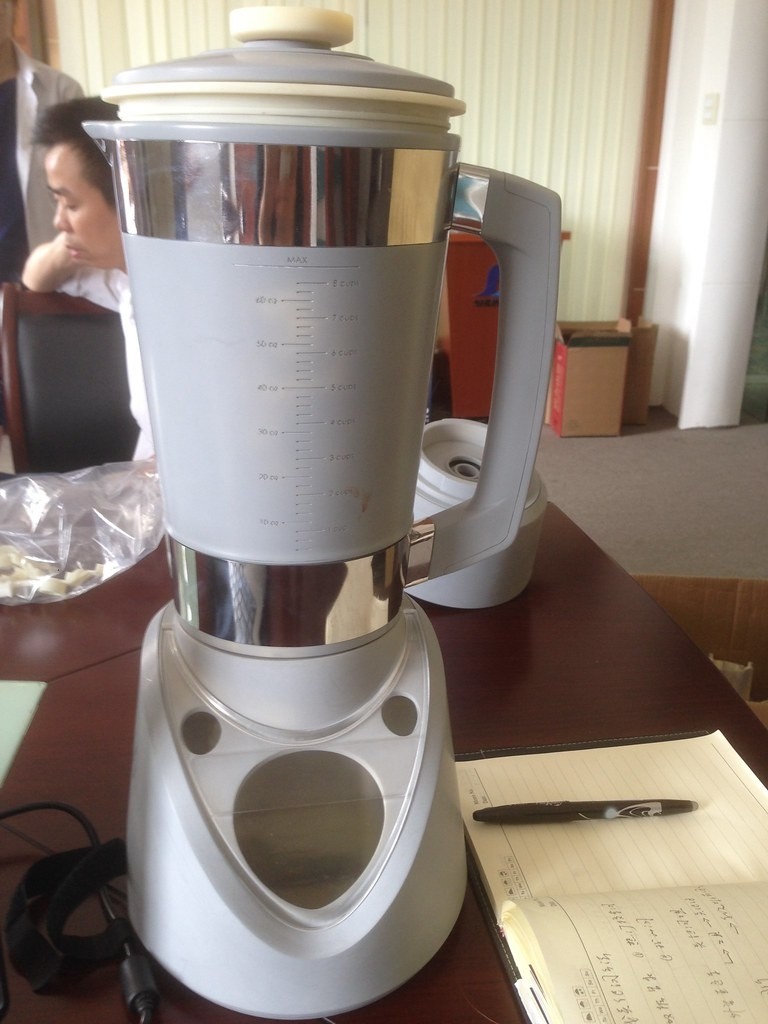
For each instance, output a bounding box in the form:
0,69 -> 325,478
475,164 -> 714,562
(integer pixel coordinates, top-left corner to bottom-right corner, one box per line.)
473,799 -> 700,826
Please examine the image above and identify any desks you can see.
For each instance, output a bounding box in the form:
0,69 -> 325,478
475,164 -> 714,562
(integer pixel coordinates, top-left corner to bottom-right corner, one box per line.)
0,416 -> 768,1024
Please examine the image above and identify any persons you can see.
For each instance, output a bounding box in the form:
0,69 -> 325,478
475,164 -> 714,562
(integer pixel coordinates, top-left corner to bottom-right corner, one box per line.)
22,97 -> 155,461
0,0 -> 85,437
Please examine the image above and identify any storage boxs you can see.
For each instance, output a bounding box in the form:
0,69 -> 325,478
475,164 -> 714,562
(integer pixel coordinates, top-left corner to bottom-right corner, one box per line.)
551,315 -> 633,435
545,316 -> 660,427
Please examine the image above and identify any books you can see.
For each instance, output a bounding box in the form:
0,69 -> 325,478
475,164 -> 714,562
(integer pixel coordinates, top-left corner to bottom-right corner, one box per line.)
455,728 -> 768,1024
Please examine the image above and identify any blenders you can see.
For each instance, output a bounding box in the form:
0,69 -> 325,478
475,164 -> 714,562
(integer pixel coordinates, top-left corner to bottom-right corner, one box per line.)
74,2 -> 562,1021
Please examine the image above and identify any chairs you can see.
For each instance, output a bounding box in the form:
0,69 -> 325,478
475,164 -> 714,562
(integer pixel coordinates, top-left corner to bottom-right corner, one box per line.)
0,281 -> 139,475
